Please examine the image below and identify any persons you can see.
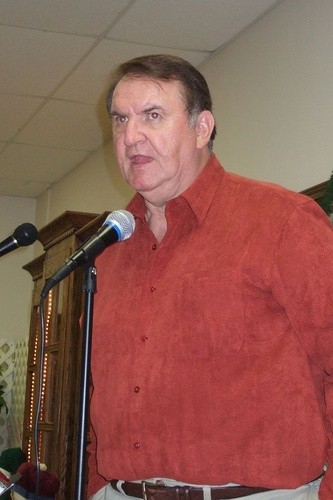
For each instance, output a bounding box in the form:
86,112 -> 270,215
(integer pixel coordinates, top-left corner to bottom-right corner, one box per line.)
85,55 -> 333,500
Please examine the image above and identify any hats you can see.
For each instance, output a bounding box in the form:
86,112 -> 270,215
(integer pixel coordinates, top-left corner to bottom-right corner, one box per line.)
0,447 -> 26,473
10,462 -> 59,500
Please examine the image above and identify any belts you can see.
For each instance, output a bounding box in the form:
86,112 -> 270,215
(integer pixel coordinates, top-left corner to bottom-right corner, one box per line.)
111,478 -> 270,500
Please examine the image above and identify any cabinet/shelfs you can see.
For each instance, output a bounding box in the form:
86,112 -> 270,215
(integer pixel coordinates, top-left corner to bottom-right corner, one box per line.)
21,212 -> 105,500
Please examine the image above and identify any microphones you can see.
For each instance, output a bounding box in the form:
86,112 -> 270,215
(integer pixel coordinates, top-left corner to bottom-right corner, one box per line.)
0,222 -> 39,257
40,209 -> 136,299
